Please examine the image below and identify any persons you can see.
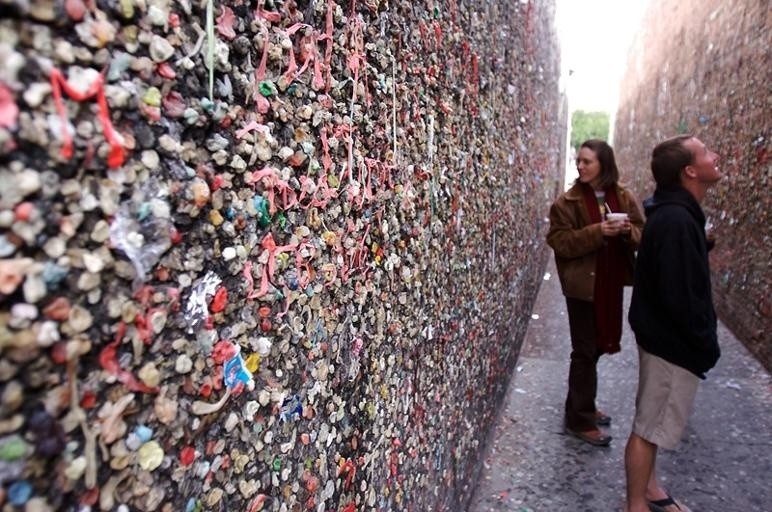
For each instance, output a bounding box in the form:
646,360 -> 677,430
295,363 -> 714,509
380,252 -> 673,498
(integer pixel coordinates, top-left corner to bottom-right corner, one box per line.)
547,137 -> 646,449
623,134 -> 723,512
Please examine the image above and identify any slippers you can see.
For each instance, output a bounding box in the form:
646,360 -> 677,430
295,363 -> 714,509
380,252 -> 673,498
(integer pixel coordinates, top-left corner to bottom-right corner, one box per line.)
646,490 -> 692,512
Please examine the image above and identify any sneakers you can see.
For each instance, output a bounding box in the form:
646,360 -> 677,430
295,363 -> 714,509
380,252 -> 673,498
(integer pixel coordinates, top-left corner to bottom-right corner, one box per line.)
595,407 -> 612,424
565,425 -> 613,446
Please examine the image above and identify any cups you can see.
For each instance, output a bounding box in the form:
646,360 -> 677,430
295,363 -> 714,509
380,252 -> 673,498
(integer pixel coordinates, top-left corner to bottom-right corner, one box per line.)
606,213 -> 628,232
